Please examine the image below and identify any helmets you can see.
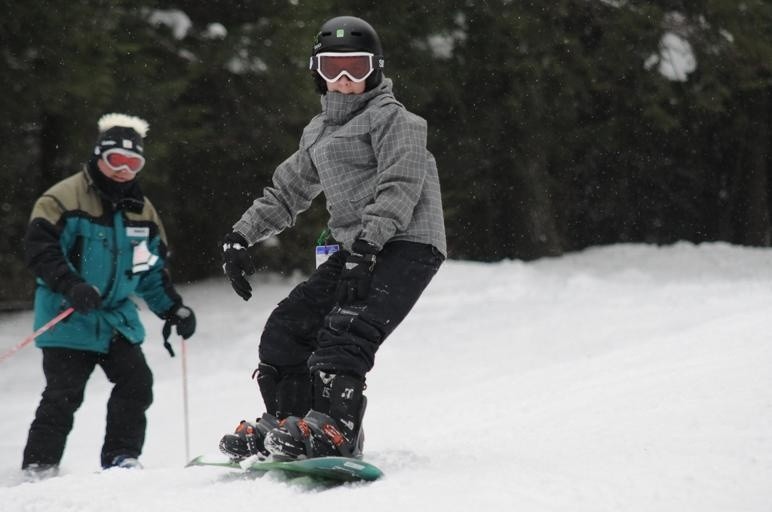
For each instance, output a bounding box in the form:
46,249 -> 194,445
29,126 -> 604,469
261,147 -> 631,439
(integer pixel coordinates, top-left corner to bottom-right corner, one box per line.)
309,15 -> 383,92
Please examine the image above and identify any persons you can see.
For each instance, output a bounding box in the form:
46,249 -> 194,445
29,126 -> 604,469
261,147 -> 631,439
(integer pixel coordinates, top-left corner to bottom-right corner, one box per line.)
20,110 -> 198,477
216,13 -> 451,465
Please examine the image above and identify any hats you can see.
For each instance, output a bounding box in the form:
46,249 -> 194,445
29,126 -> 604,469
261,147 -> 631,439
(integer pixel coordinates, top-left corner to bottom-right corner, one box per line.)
94,128 -> 145,168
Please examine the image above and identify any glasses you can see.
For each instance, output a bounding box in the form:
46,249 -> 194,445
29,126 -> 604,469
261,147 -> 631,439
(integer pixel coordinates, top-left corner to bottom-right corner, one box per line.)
102,147 -> 144,173
310,52 -> 375,84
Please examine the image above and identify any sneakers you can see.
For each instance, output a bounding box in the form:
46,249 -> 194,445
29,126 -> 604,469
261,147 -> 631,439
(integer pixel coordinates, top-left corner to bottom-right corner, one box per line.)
110,453 -> 142,468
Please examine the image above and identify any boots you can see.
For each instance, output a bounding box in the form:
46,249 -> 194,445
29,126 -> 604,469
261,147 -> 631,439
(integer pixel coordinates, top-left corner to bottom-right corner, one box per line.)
219,364 -> 313,457
265,372 -> 363,457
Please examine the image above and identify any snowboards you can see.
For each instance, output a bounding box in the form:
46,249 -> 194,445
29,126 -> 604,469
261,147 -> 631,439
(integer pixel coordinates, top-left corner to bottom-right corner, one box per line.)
190,456 -> 381,482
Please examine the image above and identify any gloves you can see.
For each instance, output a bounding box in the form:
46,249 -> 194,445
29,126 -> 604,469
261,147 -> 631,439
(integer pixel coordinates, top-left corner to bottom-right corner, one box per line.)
67,283 -> 102,315
171,305 -> 195,338
222,233 -> 253,301
335,240 -> 375,305
